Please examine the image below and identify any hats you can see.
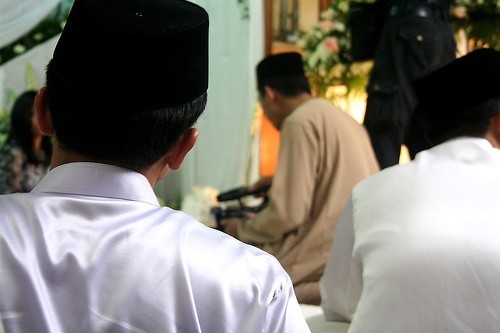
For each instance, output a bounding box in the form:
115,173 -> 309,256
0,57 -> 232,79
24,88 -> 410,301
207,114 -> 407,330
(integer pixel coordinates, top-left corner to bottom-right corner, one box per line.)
411,48 -> 500,132
257,50 -> 307,84
52,0 -> 210,110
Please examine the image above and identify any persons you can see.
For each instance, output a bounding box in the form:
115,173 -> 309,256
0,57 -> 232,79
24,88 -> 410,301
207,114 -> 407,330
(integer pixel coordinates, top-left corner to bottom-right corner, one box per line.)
349,0 -> 457,169
319,80 -> 499,333
218,50 -> 382,306
1,0 -> 307,332
1,89 -> 51,194
